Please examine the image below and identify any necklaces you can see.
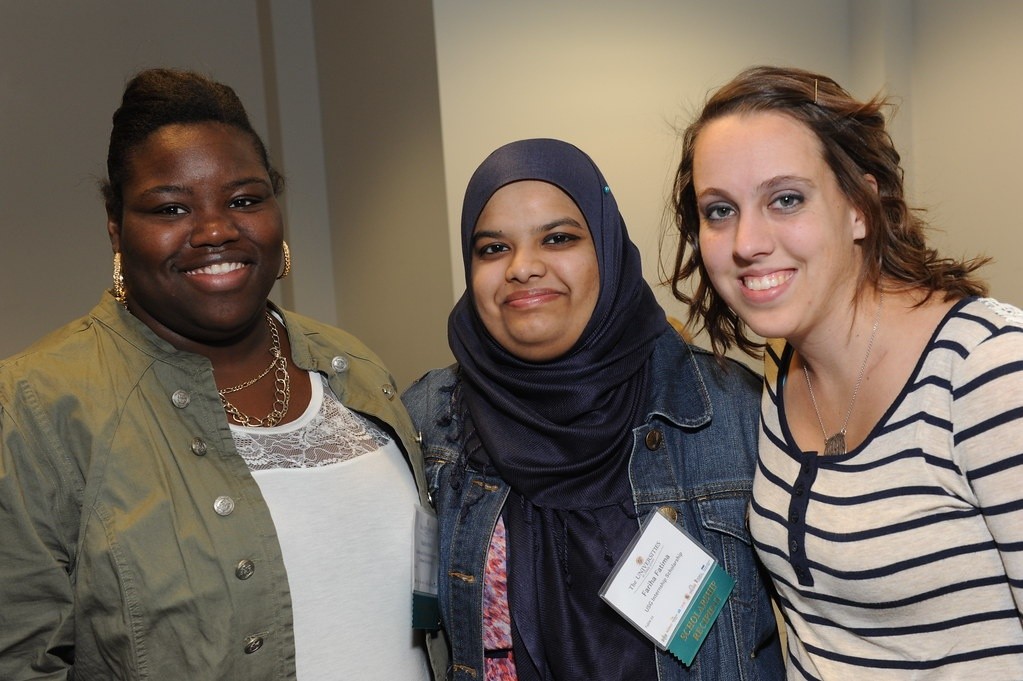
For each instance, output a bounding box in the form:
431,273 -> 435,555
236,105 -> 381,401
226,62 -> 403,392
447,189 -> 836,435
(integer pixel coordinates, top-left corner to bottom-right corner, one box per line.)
800,278 -> 883,456
218,309 -> 291,429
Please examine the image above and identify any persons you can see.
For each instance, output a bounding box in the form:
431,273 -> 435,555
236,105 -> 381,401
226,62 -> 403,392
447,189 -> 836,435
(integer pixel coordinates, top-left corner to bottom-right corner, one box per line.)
399,138 -> 788,681
0,65 -> 450,681
653,65 -> 1023,681
